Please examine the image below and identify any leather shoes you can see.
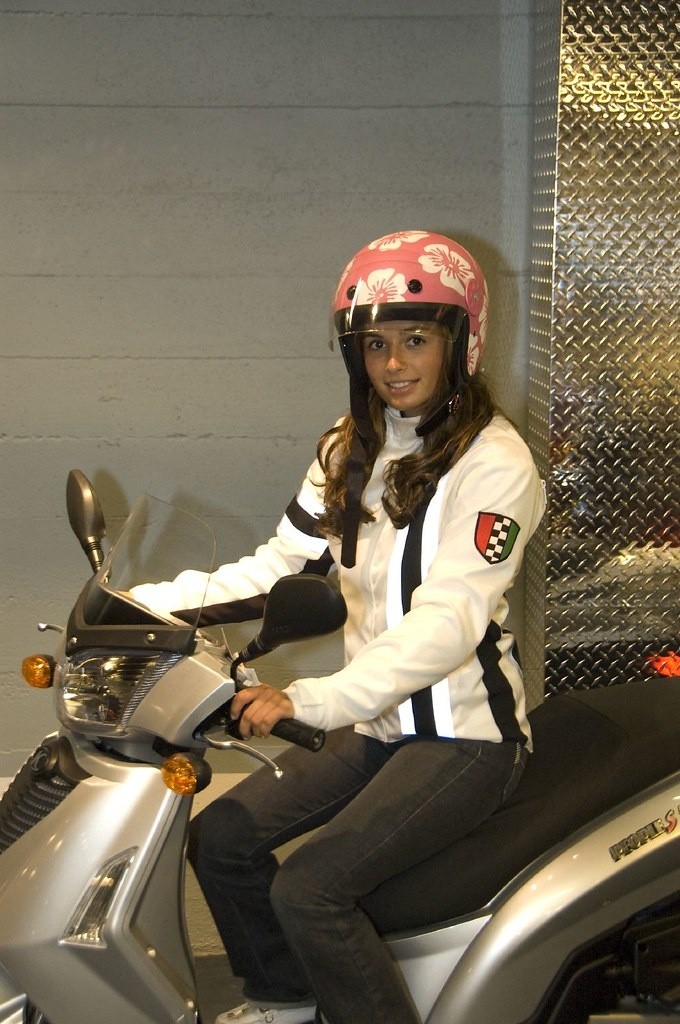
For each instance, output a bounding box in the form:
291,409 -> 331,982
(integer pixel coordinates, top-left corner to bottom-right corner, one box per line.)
215,1002 -> 317,1024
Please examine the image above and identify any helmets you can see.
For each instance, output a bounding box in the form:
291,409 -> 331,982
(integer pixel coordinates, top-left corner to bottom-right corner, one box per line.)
328,230 -> 490,376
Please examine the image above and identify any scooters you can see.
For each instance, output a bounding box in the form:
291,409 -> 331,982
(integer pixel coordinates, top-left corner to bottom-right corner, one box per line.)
0,469 -> 680,1024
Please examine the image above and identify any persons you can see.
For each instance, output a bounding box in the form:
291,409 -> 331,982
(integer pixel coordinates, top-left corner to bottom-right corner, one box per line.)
105,229 -> 545,1024
